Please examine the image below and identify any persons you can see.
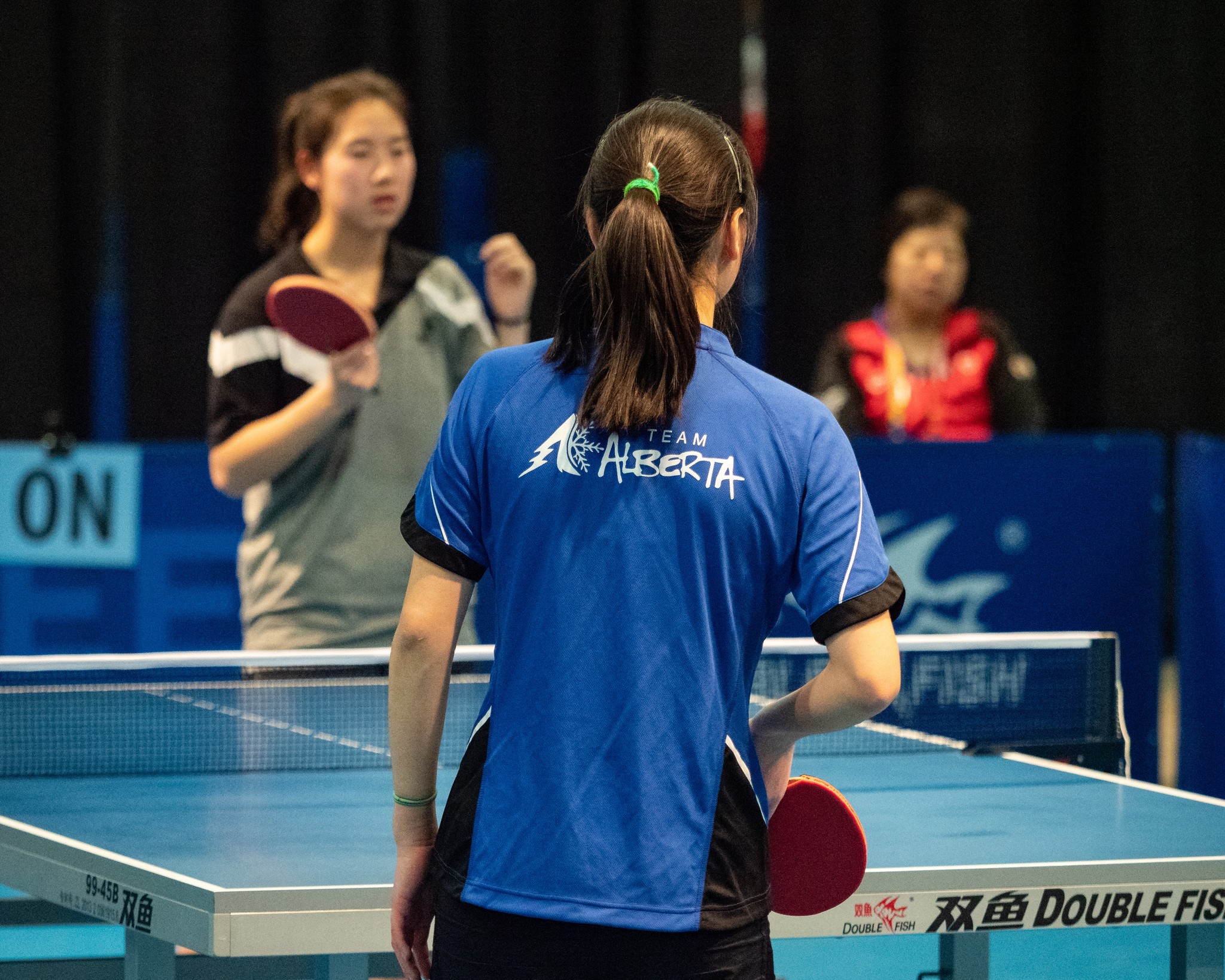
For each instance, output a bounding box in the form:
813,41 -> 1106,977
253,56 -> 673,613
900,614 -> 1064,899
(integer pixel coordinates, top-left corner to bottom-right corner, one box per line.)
812,189 -> 1042,441
207,71 -> 535,762
387,96 -> 904,980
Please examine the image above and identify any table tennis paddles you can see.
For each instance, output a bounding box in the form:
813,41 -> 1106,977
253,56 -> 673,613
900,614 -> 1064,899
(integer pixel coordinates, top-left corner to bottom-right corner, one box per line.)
769,774 -> 869,916
265,275 -> 387,389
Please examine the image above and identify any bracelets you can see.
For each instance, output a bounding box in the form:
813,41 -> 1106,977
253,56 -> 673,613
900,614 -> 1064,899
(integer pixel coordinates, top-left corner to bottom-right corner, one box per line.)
393,789 -> 437,807
496,316 -> 528,325
